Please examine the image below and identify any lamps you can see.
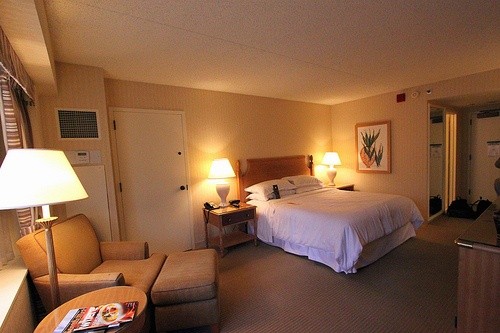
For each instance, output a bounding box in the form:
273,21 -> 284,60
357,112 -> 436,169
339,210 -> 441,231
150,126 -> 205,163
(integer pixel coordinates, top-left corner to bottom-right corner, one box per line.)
321,151 -> 341,186
208,158 -> 236,207
0,147 -> 88,307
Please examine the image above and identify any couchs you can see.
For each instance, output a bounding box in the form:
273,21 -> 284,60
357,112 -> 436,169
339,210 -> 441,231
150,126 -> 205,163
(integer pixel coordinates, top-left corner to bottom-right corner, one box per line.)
15,215 -> 168,313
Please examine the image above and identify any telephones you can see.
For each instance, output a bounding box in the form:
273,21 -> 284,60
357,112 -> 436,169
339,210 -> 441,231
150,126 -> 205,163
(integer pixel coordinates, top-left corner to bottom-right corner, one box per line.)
204,200 -> 220,210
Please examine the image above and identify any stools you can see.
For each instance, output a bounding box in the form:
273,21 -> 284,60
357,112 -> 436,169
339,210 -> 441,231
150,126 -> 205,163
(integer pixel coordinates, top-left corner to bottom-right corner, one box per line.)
150,249 -> 220,333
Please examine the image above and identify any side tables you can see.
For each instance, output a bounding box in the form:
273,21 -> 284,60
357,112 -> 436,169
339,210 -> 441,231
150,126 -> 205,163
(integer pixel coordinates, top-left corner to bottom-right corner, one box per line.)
32,286 -> 147,333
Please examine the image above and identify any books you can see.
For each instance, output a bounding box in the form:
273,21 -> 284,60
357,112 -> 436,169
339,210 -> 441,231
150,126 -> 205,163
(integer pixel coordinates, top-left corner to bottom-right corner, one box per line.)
53,301 -> 137,333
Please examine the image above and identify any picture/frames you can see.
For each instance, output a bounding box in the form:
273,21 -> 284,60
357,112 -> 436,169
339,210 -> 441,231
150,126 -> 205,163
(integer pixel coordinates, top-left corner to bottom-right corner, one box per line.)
354,121 -> 391,175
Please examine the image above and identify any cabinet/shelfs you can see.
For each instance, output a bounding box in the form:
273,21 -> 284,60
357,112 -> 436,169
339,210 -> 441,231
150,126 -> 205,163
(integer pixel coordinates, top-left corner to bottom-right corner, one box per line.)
456,196 -> 500,333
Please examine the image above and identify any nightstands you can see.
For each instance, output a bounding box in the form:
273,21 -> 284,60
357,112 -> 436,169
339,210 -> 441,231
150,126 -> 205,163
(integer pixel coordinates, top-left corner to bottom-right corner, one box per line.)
334,184 -> 355,191
200,202 -> 257,258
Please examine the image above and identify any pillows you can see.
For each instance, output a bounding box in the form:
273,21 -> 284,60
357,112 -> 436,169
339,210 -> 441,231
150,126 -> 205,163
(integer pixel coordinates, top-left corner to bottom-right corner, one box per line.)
295,185 -> 323,193
281,175 -> 322,188
245,189 -> 295,201
243,179 -> 296,196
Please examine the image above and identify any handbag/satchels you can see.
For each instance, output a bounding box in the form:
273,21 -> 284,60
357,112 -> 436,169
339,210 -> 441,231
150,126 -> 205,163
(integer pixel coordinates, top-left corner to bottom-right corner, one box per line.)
430,195 -> 442,215
447,196 -> 469,218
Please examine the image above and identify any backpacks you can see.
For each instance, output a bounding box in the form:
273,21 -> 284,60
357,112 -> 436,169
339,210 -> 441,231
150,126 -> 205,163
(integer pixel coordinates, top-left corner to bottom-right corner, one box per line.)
470,200 -> 492,219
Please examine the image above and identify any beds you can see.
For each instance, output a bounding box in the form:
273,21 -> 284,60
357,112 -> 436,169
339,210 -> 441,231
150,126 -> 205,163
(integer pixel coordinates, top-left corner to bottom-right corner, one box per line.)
238,155 -> 424,274
